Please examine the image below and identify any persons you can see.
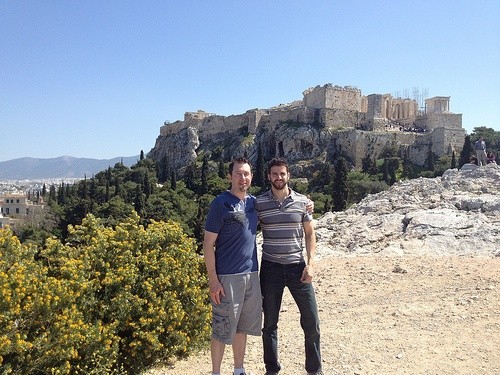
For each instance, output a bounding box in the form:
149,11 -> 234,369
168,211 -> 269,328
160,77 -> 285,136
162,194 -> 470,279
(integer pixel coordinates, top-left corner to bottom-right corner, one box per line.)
255,157 -> 325,375
203,158 -> 315,375
